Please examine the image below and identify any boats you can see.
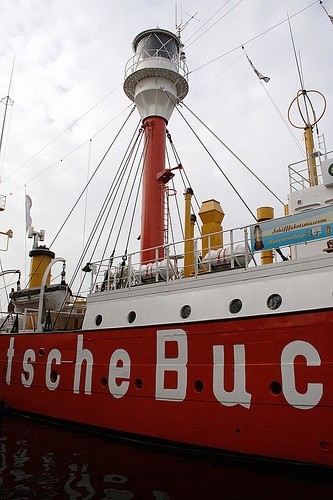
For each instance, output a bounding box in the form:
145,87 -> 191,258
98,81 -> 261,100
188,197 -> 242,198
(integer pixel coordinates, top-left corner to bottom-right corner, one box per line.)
1,0 -> 332,472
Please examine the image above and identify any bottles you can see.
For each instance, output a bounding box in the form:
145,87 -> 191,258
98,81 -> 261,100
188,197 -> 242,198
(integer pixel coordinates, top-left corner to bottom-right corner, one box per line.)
253,225 -> 264,251
